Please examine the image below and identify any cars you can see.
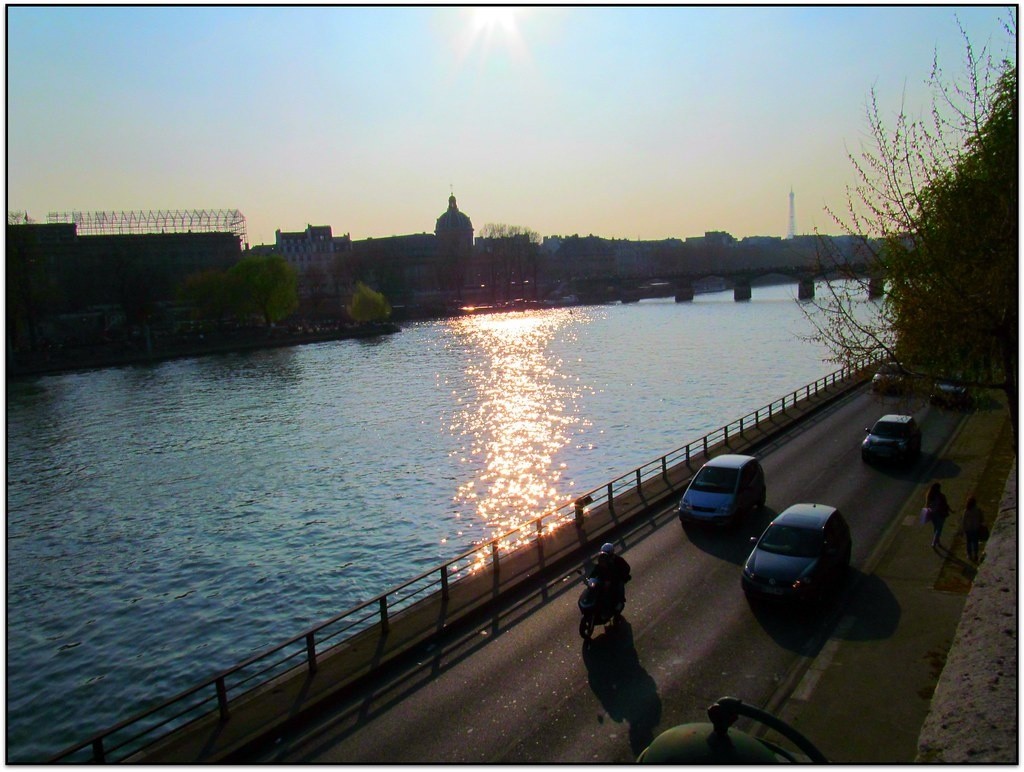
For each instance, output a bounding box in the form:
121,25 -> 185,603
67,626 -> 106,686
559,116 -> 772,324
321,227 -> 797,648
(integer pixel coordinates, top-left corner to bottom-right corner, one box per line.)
677,455 -> 766,532
873,362 -> 910,397
740,503 -> 851,609
930,368 -> 975,408
861,415 -> 922,466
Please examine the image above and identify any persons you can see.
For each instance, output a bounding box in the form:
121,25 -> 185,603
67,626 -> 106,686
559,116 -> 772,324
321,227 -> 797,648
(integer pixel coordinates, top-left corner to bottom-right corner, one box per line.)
960,498 -> 984,560
589,543 -> 630,614
927,482 -> 950,547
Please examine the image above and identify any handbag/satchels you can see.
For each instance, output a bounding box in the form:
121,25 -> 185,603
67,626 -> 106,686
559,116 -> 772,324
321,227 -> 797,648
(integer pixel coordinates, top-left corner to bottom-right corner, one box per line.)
978,525 -> 990,541
920,507 -> 933,523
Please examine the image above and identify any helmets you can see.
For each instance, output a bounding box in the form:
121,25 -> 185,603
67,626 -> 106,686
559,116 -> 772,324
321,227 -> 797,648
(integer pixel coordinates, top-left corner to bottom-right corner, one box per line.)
597,552 -> 609,567
601,543 -> 614,555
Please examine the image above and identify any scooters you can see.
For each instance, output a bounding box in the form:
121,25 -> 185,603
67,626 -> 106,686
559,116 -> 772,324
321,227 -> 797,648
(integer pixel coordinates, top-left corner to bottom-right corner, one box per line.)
576,568 -> 632,641
